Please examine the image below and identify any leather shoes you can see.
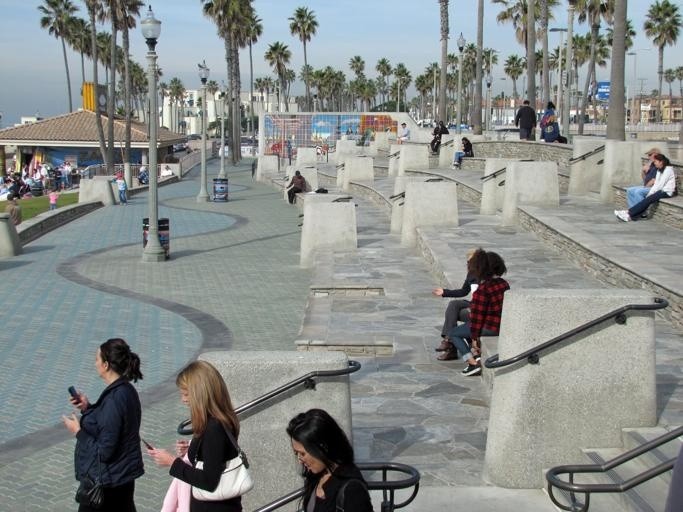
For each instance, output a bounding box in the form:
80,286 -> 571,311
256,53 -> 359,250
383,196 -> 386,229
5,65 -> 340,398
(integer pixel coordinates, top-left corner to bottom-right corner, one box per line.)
435,340 -> 453,352
437,348 -> 457,360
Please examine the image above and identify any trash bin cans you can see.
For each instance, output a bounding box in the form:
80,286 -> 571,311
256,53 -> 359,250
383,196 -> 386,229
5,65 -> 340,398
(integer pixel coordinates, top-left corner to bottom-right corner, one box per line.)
142,217 -> 169,260
212,178 -> 228,202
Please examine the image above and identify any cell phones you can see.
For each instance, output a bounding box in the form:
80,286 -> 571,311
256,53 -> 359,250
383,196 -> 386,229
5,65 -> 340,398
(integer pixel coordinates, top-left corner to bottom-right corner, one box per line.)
141,439 -> 153,449
68,386 -> 82,405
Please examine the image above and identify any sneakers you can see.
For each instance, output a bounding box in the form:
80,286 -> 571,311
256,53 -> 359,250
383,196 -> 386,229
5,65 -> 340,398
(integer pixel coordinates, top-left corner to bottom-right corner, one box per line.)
614,209 -> 626,215
460,363 -> 481,376
473,354 -> 480,362
617,214 -> 629,222
632,215 -> 648,220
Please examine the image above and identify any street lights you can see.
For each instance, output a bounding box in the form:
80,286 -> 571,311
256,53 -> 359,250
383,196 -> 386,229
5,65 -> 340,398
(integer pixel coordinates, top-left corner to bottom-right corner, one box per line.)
396,77 -> 406,113
218,80 -> 228,180
625,48 -> 651,125
141,3 -> 167,262
455,31 -> 466,136
549,28 -> 568,123
196,59 -> 212,203
482,47 -> 506,132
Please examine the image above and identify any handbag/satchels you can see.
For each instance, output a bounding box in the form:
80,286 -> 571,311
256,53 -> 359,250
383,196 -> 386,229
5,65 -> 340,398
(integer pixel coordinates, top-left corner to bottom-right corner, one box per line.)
191,415 -> 252,502
531,125 -> 535,136
75,447 -> 104,508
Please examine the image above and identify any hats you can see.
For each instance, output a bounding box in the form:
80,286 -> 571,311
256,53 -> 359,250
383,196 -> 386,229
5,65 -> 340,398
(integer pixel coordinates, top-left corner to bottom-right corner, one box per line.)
644,148 -> 660,156
116,174 -> 122,179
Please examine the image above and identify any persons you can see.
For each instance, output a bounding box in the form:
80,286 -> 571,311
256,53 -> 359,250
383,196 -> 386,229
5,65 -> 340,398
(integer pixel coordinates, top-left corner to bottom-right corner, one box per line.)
540,101 -> 568,144
627,148 -> 664,218
515,100 -> 537,139
60,338 -> 145,512
115,172 -> 128,206
430,121 -> 449,155
397,122 -> 411,144
452,137 -> 474,170
2,159 -> 76,211
435,248 -> 487,360
286,408 -> 373,512
137,166 -> 149,185
146,359 -> 251,512
286,170 -> 306,205
614,154 -> 676,222
448,252 -> 511,378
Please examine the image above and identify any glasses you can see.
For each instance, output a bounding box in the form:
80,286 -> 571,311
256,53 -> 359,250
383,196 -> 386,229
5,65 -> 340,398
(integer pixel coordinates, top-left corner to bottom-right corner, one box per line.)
292,446 -> 315,464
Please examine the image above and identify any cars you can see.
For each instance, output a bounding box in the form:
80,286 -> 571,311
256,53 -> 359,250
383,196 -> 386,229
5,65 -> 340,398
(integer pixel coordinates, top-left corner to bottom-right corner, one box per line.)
447,122 -> 473,132
173,143 -> 184,153
